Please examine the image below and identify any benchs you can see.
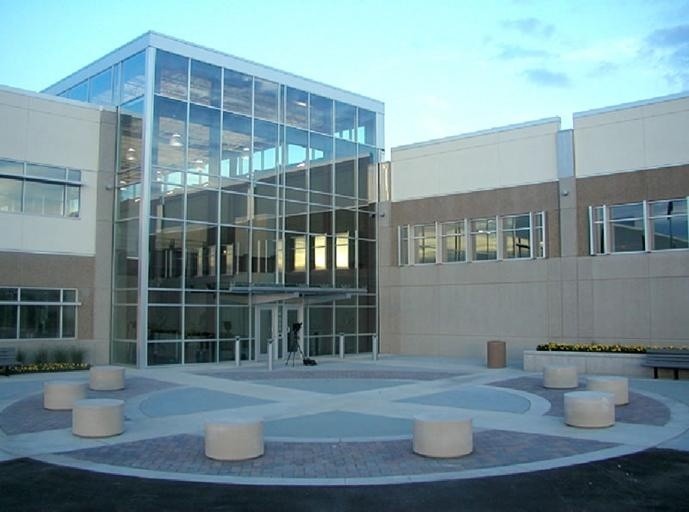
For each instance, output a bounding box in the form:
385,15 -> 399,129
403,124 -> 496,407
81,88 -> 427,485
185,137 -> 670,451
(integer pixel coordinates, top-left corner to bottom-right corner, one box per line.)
641,351 -> 689,379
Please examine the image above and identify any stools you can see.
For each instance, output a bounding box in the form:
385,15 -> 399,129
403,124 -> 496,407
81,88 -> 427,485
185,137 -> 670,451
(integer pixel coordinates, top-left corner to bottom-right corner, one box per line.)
587,376 -> 629,405
205,417 -> 264,461
564,392 -> 615,427
44,380 -> 84,410
89,366 -> 125,391
414,413 -> 473,458
72,399 -> 125,437
543,366 -> 578,388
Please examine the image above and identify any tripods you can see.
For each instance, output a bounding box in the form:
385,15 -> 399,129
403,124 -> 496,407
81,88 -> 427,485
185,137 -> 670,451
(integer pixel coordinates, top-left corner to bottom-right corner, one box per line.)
287,331 -> 306,366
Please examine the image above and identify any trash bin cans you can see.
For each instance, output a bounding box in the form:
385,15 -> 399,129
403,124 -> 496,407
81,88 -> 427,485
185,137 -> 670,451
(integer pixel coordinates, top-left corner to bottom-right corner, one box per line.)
487,341 -> 506,368
